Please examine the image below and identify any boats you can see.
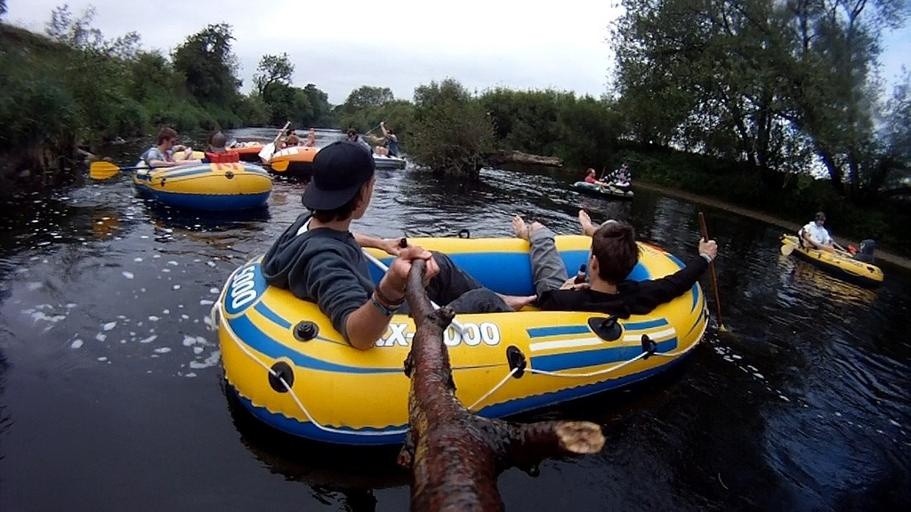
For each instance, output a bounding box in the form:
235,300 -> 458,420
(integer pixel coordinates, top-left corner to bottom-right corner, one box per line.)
271,173 -> 305,207
576,193 -> 624,213
779,254 -> 878,311
510,149 -> 562,167
217,232 -> 711,457
134,136 -> 407,211
145,205 -> 272,241
572,181 -> 634,198
779,234 -> 884,289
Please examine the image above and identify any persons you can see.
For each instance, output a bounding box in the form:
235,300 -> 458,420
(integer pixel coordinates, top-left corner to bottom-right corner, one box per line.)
513,209 -> 718,319
599,163 -> 631,186
803,212 -> 834,249
367,121 -> 400,156
842,239 -> 877,264
203,128 -> 245,158
585,168 -> 610,187
278,128 -> 315,149
258,140 -> 536,349
343,129 -> 373,155
140,127 -> 192,168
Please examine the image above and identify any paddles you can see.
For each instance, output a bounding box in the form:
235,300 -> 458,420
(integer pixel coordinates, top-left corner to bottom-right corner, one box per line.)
831,246 -> 854,258
258,120 -> 293,162
781,242 -> 799,257
89,157 -> 290,181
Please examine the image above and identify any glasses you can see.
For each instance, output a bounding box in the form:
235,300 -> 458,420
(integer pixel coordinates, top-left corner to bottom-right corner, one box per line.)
349,133 -> 355,138
292,132 -> 296,135
387,132 -> 390,134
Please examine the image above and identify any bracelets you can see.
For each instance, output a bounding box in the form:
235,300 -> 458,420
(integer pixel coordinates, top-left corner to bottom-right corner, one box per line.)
701,253 -> 713,262
373,286 -> 406,305
370,293 -> 401,319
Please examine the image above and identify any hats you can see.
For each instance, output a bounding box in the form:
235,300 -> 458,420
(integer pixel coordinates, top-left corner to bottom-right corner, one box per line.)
210,133 -> 227,154
814,211 -> 826,221
301,140 -> 376,212
